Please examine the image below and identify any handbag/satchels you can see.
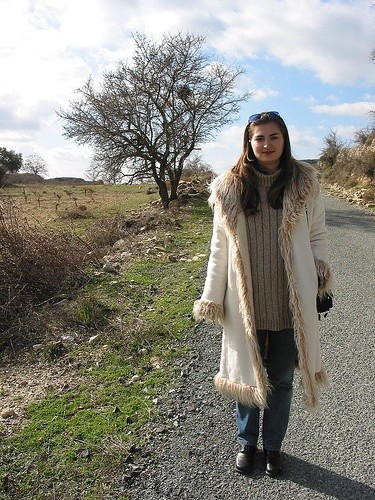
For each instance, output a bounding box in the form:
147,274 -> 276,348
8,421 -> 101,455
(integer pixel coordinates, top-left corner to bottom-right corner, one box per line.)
316,291 -> 333,314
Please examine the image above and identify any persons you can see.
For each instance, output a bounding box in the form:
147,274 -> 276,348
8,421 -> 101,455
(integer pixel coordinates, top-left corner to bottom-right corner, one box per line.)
192,111 -> 333,476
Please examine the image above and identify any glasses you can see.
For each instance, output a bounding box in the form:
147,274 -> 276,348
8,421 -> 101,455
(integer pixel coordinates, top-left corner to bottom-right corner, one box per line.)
248,111 -> 279,122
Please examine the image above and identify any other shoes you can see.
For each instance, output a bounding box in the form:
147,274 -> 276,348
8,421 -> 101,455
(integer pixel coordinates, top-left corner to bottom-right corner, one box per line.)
265,449 -> 282,476
234,445 -> 257,474
